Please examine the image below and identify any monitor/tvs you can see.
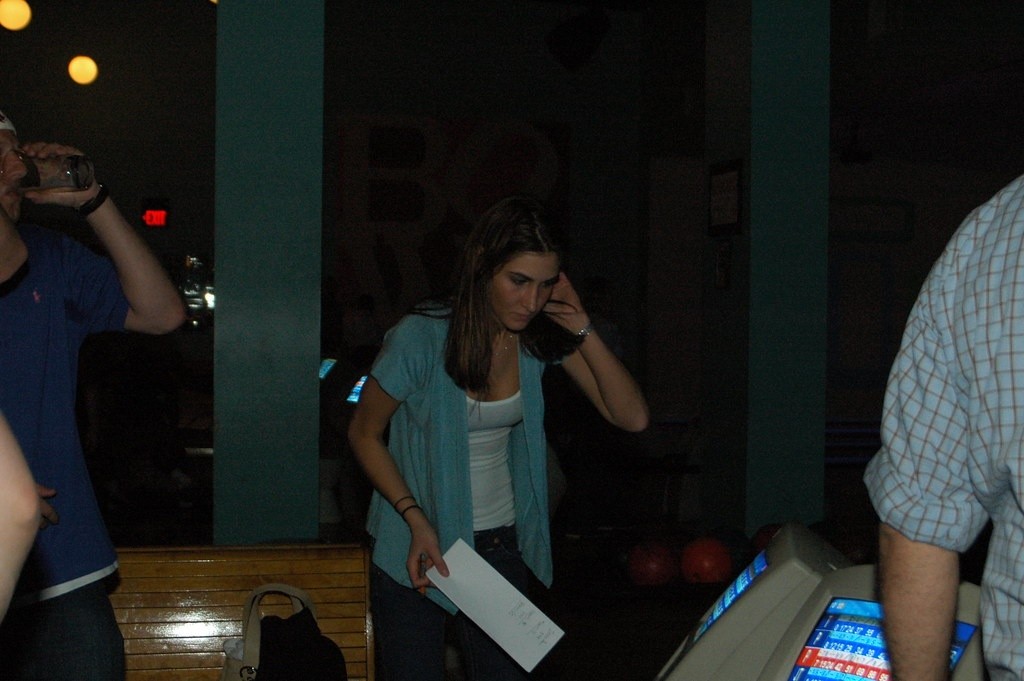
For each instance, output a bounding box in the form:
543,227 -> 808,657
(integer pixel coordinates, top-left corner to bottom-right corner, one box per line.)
320,359 -> 371,402
647,522 -> 991,681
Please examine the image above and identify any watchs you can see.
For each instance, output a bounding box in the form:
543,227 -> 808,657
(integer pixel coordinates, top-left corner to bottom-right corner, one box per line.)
78,186 -> 110,216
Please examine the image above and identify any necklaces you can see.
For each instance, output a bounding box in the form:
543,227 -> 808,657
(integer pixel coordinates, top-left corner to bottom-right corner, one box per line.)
491,335 -> 513,356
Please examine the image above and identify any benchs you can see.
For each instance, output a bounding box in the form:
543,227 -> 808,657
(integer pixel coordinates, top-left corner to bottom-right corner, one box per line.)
108,543 -> 376,681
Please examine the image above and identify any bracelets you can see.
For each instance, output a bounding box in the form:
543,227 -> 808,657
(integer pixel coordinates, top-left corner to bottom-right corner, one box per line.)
401,505 -> 421,516
394,496 -> 416,508
579,323 -> 594,338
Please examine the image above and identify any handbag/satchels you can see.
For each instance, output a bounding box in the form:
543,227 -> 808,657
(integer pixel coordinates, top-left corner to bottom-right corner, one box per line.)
219,583 -> 317,681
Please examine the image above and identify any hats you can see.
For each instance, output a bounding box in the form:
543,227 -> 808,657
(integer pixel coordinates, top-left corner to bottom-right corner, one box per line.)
0,111 -> 17,135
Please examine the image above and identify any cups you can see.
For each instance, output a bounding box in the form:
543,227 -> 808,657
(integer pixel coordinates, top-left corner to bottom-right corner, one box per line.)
0,148 -> 94,191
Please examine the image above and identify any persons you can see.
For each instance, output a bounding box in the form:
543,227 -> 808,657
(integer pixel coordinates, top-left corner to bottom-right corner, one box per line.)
0,109 -> 185,681
863,175 -> 1024,681
346,196 -> 648,681
0,415 -> 40,620
583,275 -> 623,361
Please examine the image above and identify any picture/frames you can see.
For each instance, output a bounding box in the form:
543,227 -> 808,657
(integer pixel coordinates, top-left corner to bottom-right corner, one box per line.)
705,158 -> 744,236
